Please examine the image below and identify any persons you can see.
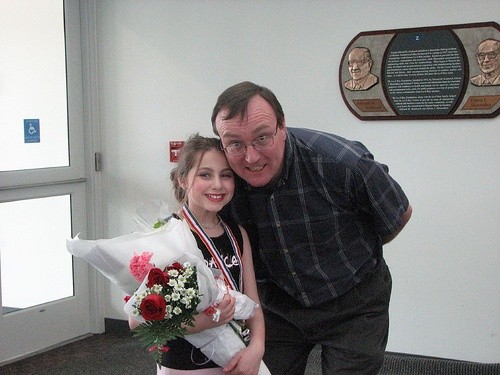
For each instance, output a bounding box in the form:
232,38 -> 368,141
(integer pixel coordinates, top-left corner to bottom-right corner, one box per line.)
211,82 -> 413,375
128,137 -> 273,375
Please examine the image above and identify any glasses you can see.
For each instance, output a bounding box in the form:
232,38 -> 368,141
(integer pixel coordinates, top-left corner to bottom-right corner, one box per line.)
220,121 -> 279,157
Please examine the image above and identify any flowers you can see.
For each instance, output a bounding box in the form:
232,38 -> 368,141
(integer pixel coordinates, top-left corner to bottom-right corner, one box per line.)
69,201 -> 272,375
123,253 -> 259,370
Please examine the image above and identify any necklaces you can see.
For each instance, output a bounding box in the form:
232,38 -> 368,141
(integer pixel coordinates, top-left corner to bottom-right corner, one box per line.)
193,218 -> 225,229
